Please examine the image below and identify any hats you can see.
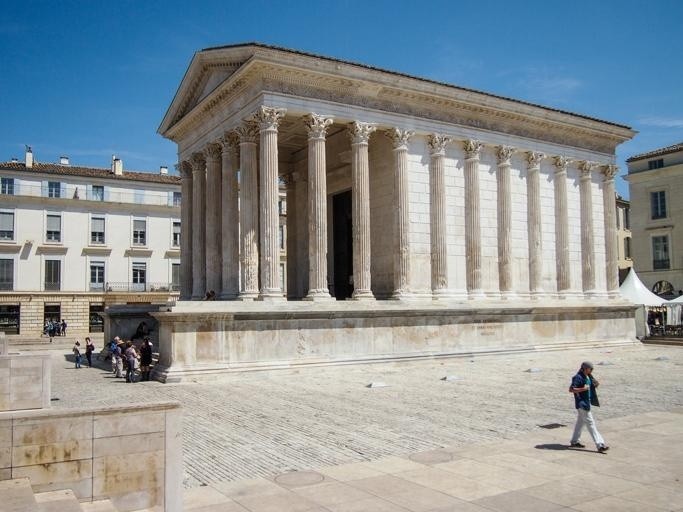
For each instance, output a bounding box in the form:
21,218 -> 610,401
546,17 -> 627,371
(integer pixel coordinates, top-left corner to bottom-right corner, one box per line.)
116,339 -> 125,346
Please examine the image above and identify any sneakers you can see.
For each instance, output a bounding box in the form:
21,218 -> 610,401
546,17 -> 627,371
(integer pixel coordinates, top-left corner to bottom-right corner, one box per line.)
598,446 -> 609,452
569,443 -> 586,448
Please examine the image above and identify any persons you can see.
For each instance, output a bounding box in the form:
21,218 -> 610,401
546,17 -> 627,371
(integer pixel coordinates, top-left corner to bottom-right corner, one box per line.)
110,335 -> 152,384
72,341 -> 82,369
85,337 -> 94,367
43,319 -> 66,337
569,361 -> 610,452
